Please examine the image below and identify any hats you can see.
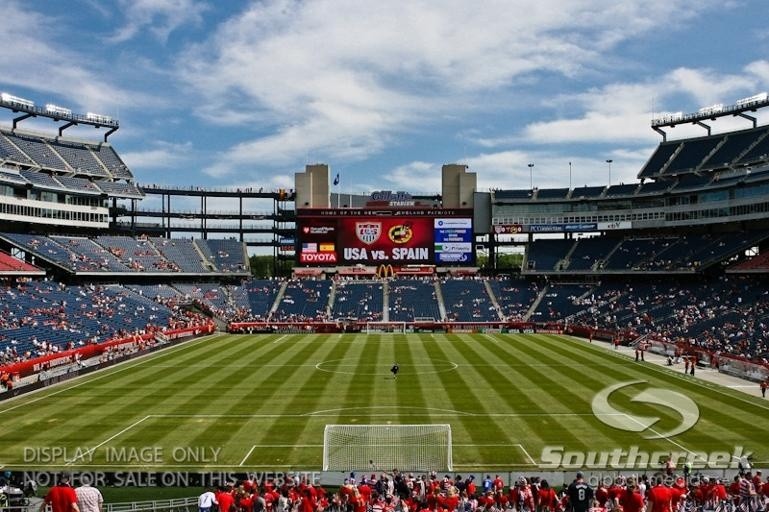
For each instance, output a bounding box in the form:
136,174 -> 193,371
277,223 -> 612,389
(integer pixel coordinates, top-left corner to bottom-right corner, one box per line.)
26,479 -> 39,491
80,475 -> 91,484
58,469 -> 73,479
200,469 -> 769,501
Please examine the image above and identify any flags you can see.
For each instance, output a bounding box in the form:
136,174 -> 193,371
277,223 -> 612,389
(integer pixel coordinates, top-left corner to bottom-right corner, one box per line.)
333,174 -> 339,185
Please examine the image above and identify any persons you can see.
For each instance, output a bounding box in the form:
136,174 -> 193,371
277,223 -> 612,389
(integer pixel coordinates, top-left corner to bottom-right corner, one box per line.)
0,469 -> 104,512
2,181 -> 768,396
199,454 -> 768,512
